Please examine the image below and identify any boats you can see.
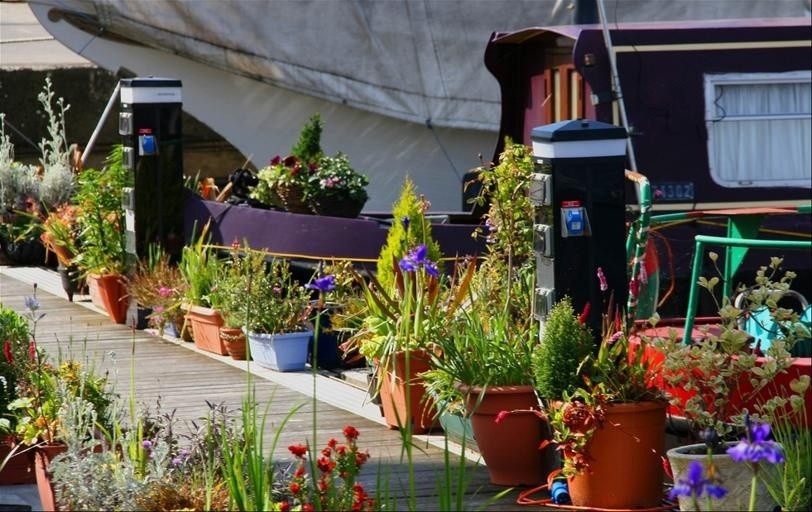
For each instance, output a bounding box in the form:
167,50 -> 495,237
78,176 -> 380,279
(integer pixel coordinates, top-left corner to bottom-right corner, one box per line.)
181,18 -> 812,357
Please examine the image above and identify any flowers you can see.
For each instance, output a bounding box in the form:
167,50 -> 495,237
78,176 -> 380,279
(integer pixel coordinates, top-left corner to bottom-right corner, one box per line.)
0,74 -> 812,512
